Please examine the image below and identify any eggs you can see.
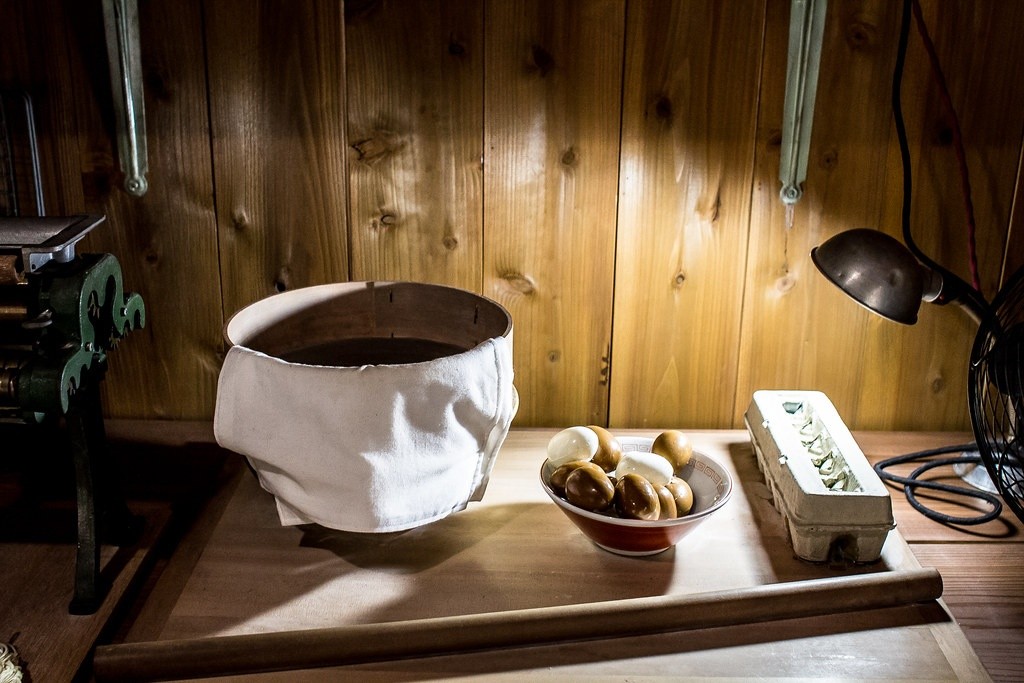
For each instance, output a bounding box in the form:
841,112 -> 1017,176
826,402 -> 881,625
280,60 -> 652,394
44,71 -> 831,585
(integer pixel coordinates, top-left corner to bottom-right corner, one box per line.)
547,426 -> 694,521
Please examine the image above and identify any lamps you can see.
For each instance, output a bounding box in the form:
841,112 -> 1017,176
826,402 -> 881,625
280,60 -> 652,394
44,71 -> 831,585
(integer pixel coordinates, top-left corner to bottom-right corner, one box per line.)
811,228 -> 1007,346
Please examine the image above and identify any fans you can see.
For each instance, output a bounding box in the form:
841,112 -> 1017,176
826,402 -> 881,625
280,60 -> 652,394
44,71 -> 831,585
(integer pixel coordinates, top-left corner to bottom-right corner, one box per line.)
968,265 -> 1024,525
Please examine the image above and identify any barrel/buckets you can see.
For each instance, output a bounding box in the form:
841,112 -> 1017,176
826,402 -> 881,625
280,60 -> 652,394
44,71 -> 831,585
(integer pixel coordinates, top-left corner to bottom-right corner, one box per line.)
222,280 -> 515,543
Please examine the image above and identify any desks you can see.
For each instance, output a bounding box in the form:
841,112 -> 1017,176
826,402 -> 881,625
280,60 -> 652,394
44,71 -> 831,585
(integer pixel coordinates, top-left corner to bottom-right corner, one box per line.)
104,418 -> 1024,683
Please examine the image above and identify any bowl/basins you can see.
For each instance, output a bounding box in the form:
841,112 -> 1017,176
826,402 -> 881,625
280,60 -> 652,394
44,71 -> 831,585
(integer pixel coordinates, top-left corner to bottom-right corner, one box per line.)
539,435 -> 734,557
